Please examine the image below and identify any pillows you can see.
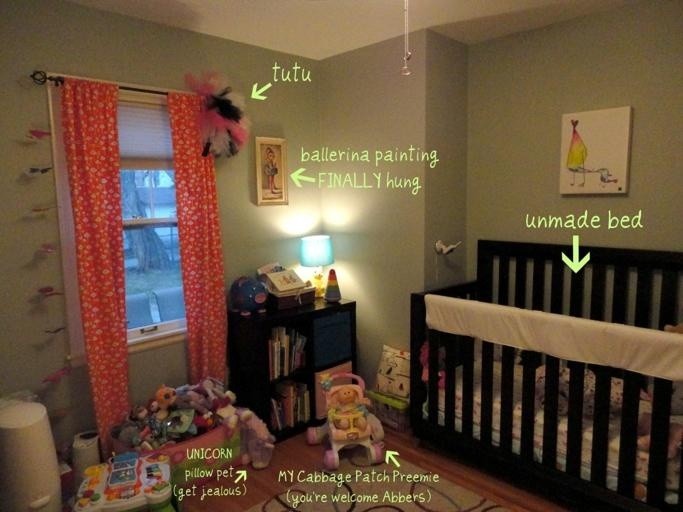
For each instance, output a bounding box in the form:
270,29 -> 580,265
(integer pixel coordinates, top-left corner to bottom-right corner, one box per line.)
374,344 -> 410,398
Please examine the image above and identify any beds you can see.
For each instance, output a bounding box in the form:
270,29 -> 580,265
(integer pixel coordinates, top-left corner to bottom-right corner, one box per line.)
413,239 -> 683,512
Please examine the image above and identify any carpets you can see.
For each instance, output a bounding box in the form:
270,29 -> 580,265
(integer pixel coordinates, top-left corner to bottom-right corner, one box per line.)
245,448 -> 510,512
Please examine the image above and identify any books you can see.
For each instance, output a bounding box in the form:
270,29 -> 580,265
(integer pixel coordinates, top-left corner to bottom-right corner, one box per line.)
262,322 -> 312,443
263,266 -> 307,298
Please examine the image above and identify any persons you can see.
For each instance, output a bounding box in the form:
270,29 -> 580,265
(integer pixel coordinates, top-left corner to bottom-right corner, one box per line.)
299,369 -> 386,470
70,447 -> 176,512
321,270 -> 341,304
228,273 -> 270,317
102,375 -> 275,482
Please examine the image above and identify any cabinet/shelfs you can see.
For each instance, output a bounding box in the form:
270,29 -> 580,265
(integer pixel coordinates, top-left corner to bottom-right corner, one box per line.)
228,297 -> 356,443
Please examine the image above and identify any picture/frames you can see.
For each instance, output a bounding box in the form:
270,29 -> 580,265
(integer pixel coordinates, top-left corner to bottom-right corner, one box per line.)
255,136 -> 288,206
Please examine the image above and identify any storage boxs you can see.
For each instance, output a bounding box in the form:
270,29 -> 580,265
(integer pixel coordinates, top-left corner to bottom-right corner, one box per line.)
366,390 -> 412,432
110,420 -> 241,496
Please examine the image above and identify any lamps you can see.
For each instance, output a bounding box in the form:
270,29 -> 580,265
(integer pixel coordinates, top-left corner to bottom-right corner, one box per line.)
402,0 -> 411,75
300,235 -> 333,299
